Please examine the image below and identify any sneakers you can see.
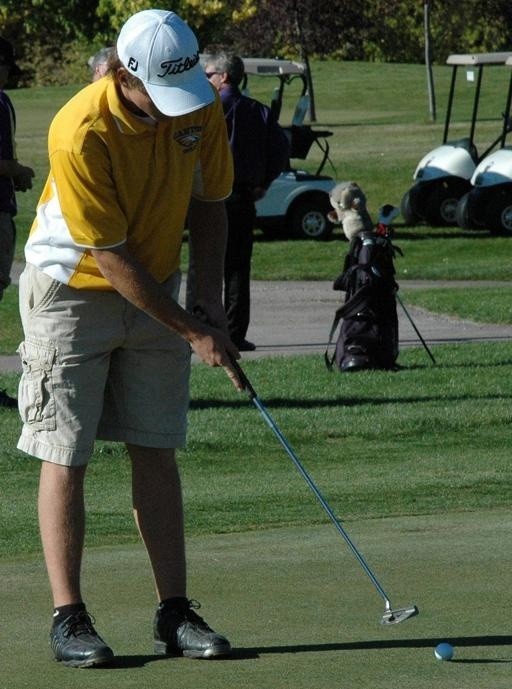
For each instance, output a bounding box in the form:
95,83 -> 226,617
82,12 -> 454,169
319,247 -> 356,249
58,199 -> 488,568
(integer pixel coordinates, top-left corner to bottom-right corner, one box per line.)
49,608 -> 114,668
152,603 -> 231,658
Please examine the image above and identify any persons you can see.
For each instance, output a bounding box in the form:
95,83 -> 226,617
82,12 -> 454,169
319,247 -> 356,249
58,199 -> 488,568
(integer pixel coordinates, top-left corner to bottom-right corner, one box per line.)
188,52 -> 289,351
19,10 -> 241,667
2,32 -> 34,410
89,46 -> 113,82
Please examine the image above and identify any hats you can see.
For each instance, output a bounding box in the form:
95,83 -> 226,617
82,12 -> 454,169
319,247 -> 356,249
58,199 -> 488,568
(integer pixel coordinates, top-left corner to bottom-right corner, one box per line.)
116,10 -> 216,118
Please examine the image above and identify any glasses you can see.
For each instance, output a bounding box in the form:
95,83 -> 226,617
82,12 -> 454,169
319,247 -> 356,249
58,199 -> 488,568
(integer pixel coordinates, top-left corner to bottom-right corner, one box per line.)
205,72 -> 219,79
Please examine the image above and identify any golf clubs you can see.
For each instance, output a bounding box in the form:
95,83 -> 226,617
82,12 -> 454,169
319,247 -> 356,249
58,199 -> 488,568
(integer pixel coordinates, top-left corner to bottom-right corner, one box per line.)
370,267 -> 441,365
188,304 -> 419,626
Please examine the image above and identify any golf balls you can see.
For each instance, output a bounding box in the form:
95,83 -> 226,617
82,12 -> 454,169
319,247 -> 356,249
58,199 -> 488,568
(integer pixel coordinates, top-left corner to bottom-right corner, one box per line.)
434,643 -> 454,661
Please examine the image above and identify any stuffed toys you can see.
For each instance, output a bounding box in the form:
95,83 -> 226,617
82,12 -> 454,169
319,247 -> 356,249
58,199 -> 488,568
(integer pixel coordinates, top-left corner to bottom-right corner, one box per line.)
328,180 -> 374,241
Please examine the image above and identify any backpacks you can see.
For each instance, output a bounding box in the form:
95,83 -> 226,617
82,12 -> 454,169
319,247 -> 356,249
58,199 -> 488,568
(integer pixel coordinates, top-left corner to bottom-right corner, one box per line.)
324,223 -> 404,372
